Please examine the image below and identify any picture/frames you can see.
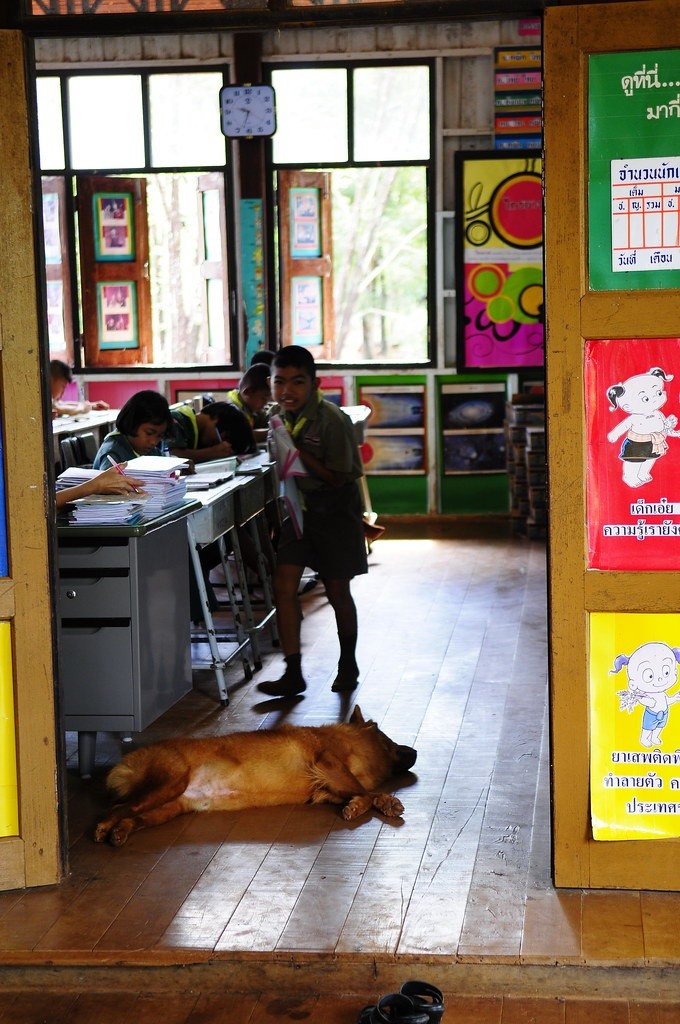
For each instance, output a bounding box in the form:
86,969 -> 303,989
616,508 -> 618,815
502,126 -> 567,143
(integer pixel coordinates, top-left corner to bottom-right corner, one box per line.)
95,281 -> 138,350
93,191 -> 135,262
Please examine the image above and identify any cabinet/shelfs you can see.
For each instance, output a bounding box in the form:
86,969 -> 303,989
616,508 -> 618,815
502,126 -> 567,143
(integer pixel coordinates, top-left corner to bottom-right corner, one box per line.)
57,498 -> 203,779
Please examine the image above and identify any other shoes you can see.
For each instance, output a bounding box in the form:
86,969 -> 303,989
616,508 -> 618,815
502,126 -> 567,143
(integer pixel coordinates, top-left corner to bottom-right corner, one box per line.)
331,659 -> 359,693
257,673 -> 307,696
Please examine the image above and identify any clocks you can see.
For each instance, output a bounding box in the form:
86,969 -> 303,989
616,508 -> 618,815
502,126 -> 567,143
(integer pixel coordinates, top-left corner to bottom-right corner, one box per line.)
219,83 -> 277,140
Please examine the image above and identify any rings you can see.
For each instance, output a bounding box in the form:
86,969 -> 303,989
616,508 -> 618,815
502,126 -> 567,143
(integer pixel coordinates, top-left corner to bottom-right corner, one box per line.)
127,493 -> 130,496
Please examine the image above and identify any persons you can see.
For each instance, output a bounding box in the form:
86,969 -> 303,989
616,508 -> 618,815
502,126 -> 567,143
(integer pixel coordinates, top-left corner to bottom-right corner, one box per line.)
50,360 -> 109,418
226,349 -> 275,443
93,390 -> 219,621
159,396 -> 317,599
257,345 -> 368,697
55,461 -> 147,510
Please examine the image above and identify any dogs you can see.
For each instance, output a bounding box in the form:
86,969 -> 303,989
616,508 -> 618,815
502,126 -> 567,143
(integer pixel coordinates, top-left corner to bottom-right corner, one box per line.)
93,705 -> 418,847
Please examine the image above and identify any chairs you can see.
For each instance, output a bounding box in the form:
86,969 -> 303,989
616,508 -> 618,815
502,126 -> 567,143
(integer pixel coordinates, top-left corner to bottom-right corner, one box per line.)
60,432 -> 97,469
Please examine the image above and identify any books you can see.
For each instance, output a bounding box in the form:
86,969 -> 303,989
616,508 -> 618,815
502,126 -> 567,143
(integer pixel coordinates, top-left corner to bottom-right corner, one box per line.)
56,451 -> 262,525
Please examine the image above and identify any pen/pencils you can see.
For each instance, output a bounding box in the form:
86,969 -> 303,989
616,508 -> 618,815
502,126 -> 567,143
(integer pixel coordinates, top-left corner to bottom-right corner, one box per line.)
215,427 -> 222,443
106,454 -> 141,494
79,381 -> 85,401
163,445 -> 169,457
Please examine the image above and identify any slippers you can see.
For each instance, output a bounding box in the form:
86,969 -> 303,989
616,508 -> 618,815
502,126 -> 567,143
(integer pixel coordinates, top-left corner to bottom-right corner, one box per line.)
358,980 -> 445,1024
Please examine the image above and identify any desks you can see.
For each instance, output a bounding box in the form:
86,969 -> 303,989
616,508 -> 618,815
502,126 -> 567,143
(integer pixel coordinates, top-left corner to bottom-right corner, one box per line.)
53,404 -> 371,704
